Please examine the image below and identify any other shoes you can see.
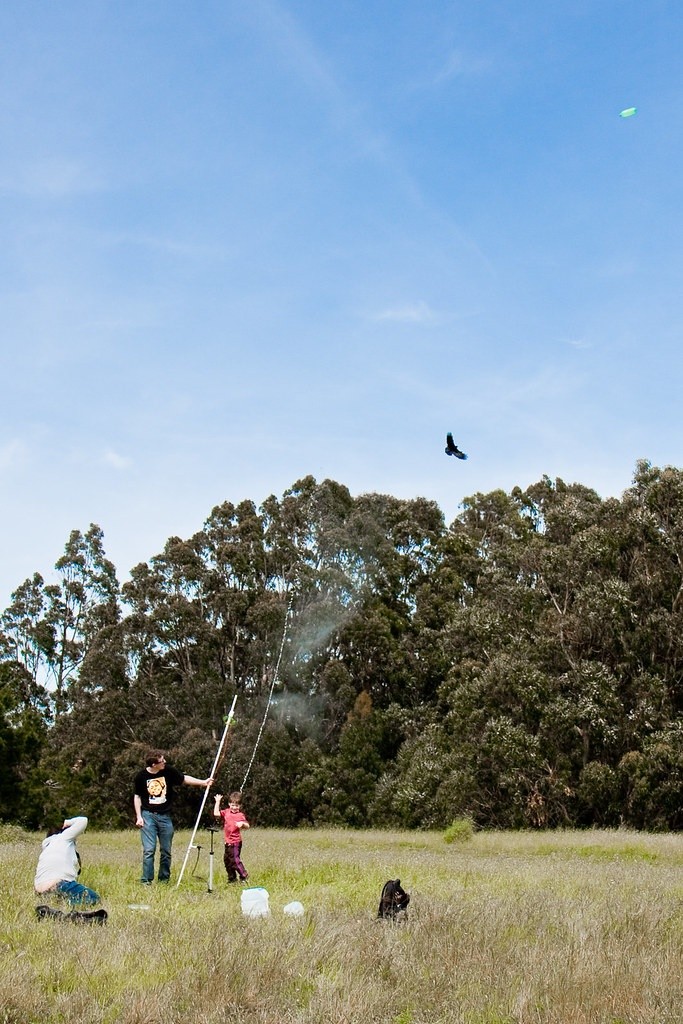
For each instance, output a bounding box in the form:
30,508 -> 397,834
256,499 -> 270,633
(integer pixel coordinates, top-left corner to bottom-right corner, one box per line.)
227,878 -> 239,885
158,877 -> 170,884
140,879 -> 153,886
238,875 -> 250,884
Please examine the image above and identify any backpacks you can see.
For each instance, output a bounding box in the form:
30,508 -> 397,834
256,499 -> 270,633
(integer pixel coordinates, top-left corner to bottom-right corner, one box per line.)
377,879 -> 410,924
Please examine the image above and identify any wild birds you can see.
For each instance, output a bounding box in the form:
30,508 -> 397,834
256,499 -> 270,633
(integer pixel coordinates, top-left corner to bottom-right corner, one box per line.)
445,432 -> 468,460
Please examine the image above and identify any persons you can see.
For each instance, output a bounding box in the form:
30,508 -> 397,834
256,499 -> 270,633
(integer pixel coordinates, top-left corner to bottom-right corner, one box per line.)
34,816 -> 100,905
133,749 -> 213,886
214,792 -> 250,885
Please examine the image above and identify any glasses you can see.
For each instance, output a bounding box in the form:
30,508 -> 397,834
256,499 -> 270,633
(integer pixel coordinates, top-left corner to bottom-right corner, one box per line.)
155,758 -> 165,764
